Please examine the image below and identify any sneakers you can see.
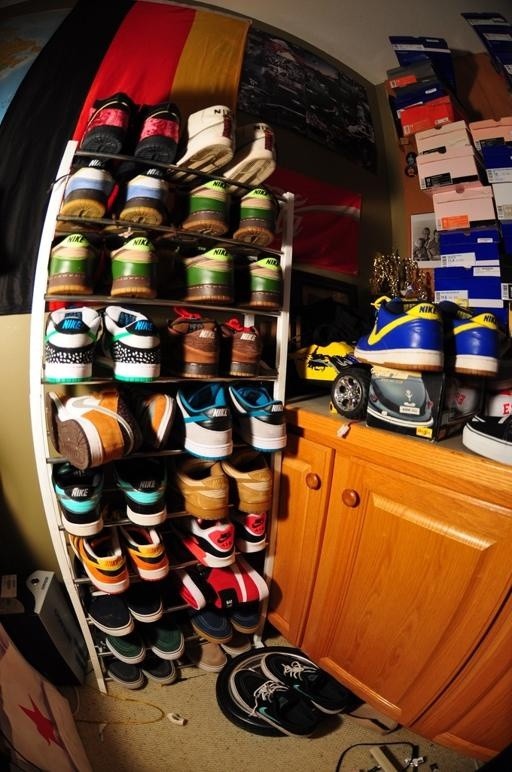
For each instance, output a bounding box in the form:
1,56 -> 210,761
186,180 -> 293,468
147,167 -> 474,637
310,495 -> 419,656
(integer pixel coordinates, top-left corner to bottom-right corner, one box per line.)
462,414 -> 512,466
439,300 -> 500,376
230,669 -> 323,738
261,652 -> 349,714
353,296 -> 445,371
42,93 -> 287,689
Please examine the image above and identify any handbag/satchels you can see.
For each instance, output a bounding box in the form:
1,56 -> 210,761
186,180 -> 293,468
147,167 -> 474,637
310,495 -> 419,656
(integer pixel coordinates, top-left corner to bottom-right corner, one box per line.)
1,640 -> 92,772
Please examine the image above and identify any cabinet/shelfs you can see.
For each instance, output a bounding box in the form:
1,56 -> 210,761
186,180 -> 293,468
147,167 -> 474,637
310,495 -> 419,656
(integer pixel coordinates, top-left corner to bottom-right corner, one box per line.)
25,135 -> 298,698
266,392 -> 512,764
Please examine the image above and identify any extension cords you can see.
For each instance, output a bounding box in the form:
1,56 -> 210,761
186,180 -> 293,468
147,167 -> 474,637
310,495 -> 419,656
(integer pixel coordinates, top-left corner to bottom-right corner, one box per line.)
369,746 -> 399,772
